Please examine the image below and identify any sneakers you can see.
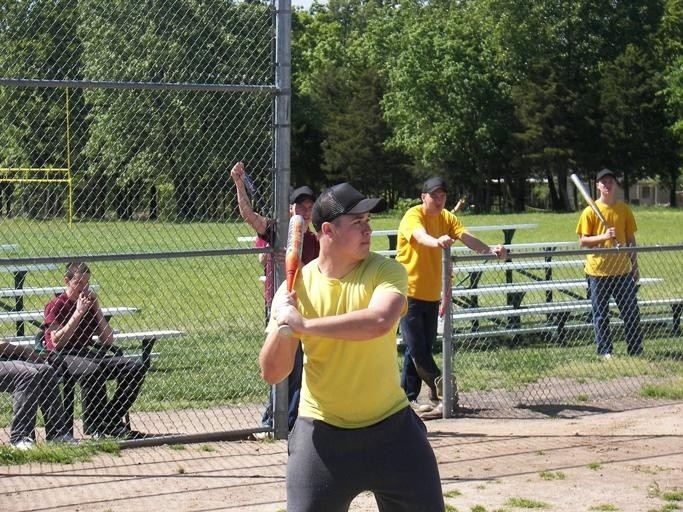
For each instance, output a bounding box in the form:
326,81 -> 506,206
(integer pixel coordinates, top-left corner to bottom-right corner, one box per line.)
9,421 -> 139,451
408,398 -> 457,419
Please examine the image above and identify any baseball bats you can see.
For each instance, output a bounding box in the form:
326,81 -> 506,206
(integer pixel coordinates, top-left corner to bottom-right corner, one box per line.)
570,172 -> 619,246
285,216 -> 305,294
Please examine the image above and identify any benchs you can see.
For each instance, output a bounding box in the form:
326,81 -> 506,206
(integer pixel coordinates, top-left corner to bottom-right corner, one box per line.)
1,244 -> 188,424
235,223 -> 683,348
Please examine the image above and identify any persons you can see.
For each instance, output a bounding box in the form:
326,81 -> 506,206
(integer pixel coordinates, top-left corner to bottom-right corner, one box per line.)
576,168 -> 647,361
394,176 -> 508,413
257,182 -> 446,512
43,263 -> 148,441
229,161 -> 320,432
450,197 -> 466,213
0,342 -> 86,453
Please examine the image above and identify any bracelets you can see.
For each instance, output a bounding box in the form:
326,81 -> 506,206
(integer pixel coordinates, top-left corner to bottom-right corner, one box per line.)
453,207 -> 457,213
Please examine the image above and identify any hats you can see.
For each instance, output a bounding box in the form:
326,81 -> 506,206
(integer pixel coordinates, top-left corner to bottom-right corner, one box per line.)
595,168 -> 616,182
287,186 -> 316,205
421,176 -> 448,195
310,183 -> 386,232
